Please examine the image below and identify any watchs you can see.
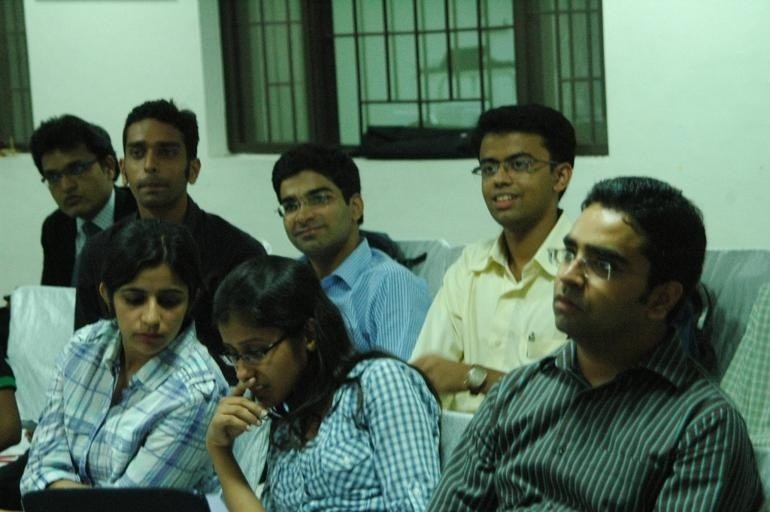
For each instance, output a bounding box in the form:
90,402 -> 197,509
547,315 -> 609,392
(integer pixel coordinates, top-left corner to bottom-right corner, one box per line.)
462,360 -> 488,397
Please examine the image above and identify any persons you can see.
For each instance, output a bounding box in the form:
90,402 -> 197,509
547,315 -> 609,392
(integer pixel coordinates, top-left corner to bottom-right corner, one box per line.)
405,102 -> 577,414
205,254 -> 444,511
269,142 -> 433,364
425,170 -> 766,511
71,92 -> 269,391
28,113 -> 141,286
19,218 -> 229,511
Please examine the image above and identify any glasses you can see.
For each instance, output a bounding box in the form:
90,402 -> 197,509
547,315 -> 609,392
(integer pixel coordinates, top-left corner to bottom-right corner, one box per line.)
219,328 -> 300,366
549,248 -> 655,281
42,157 -> 100,184
472,156 -> 556,175
278,192 -> 329,218
